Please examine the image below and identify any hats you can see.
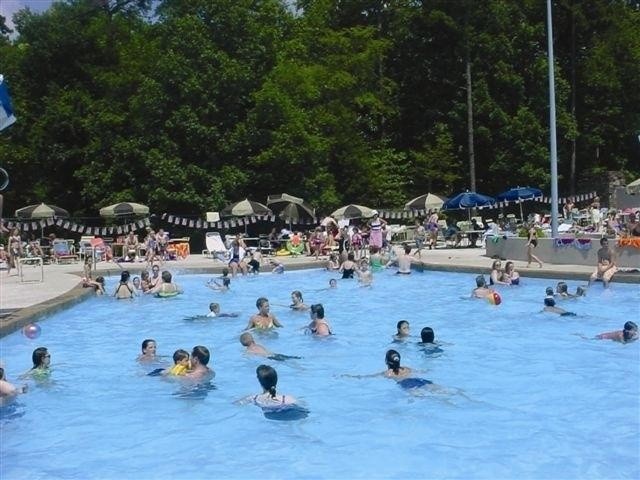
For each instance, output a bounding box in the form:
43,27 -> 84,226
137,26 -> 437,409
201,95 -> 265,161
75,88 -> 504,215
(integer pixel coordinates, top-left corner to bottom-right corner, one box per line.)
371,209 -> 378,217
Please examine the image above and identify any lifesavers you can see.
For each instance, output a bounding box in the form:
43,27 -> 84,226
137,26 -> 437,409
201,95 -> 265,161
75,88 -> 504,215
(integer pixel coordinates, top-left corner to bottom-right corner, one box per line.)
286,238 -> 305,254
168,364 -> 187,376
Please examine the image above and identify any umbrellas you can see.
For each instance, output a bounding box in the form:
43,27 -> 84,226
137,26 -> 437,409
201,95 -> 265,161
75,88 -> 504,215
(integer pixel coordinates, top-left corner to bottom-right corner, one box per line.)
100,201 -> 149,237
328,203 -> 378,234
445,190 -> 494,231
626,178 -> 640,195
494,185 -> 543,224
15,202 -> 71,238
403,191 -> 446,224
265,193 -> 317,238
219,198 -> 273,238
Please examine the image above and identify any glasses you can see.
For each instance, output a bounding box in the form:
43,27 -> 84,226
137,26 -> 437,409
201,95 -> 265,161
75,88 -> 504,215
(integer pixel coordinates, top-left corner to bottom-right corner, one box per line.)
44,354 -> 51,358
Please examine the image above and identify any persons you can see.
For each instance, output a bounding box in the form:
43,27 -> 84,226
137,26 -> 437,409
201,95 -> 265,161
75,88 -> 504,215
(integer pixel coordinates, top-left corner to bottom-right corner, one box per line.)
445,197 -> 639,316
25,347 -> 52,381
207,302 -> 221,318
245,366 -> 305,417
391,320 -> 413,344
292,212 -> 439,291
184,345 -> 215,387
0,368 -> 23,407
244,297 -> 283,336
135,339 -> 164,365
594,320 -> 640,344
207,229 -> 285,292
376,349 -> 448,397
239,332 -> 298,364
1,218 -> 184,301
281,292 -> 309,312
147,350 -> 190,380
298,303 -> 332,337
416,327 -> 444,355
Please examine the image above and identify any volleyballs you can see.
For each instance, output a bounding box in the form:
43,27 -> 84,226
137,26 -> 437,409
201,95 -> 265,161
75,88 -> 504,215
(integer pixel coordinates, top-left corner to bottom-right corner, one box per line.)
24,324 -> 41,339
484,292 -> 502,306
